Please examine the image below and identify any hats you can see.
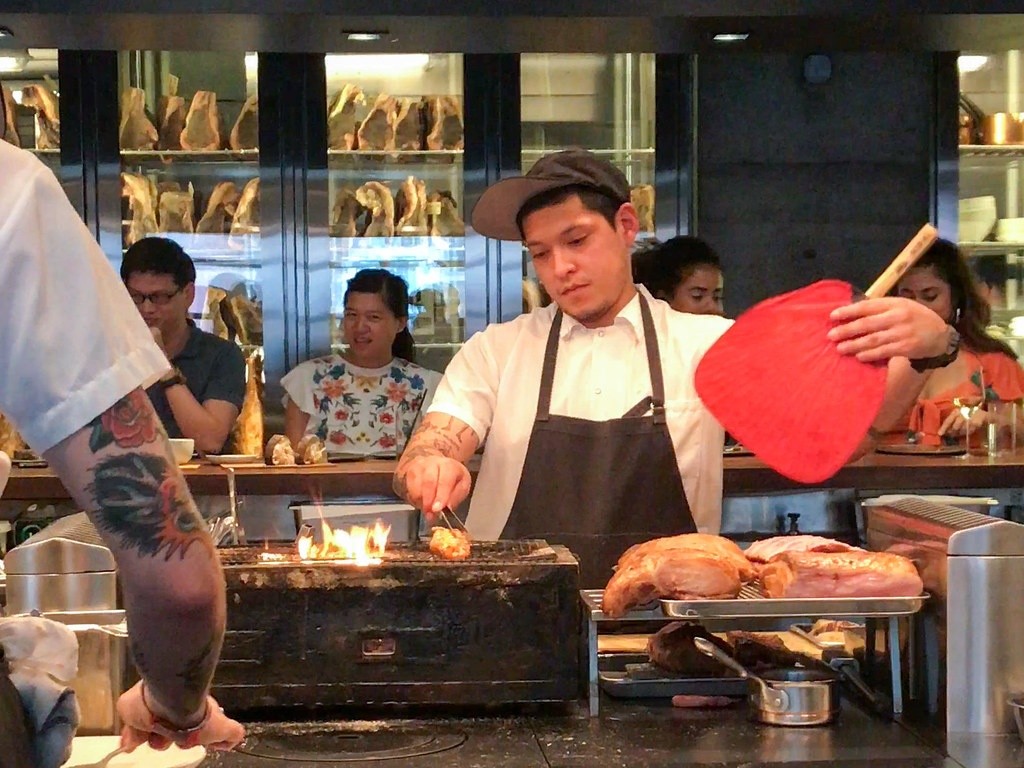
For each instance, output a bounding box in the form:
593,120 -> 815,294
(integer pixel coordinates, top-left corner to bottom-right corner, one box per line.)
470,149 -> 631,241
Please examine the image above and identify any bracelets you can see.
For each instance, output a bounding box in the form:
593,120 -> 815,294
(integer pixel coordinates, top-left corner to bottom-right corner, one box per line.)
141,679 -> 211,736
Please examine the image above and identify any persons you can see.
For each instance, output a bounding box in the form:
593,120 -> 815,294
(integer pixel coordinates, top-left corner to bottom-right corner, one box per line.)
0,139 -> 243,768
393,150 -> 959,591
632,234 -> 726,315
119,237 -> 247,454
898,238 -> 1024,452
281,269 -> 443,461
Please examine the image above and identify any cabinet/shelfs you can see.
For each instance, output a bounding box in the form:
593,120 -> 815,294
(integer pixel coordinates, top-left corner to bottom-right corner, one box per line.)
0,53 -> 697,410
938,52 -> 1024,341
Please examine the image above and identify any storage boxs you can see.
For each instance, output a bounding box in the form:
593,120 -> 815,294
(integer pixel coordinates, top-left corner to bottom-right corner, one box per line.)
290,497 -> 423,543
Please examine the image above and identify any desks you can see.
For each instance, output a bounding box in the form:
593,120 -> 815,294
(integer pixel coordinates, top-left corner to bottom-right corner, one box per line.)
1,447 -> 1024,554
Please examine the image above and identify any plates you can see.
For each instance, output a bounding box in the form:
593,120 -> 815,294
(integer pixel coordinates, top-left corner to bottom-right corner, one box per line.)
205,454 -> 261,465
57,735 -> 206,768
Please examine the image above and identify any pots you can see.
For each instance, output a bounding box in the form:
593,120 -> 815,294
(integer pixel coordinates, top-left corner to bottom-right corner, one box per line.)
693,635 -> 840,725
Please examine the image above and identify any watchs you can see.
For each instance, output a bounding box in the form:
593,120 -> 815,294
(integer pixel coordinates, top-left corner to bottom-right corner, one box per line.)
160,369 -> 184,388
908,325 -> 960,372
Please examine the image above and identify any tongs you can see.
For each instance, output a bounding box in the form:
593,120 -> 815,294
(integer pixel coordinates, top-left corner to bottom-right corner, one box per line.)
439,505 -> 471,540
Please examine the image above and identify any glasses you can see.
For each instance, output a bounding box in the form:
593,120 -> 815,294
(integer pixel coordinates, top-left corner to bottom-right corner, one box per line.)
126,280 -> 195,305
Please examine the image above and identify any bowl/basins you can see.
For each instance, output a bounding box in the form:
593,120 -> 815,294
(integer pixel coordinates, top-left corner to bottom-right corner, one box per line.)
959,112 -> 1024,145
959,195 -> 1024,244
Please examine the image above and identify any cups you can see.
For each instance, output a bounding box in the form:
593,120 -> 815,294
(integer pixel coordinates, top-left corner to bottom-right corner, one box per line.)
168,438 -> 195,465
965,399 -> 1016,460
